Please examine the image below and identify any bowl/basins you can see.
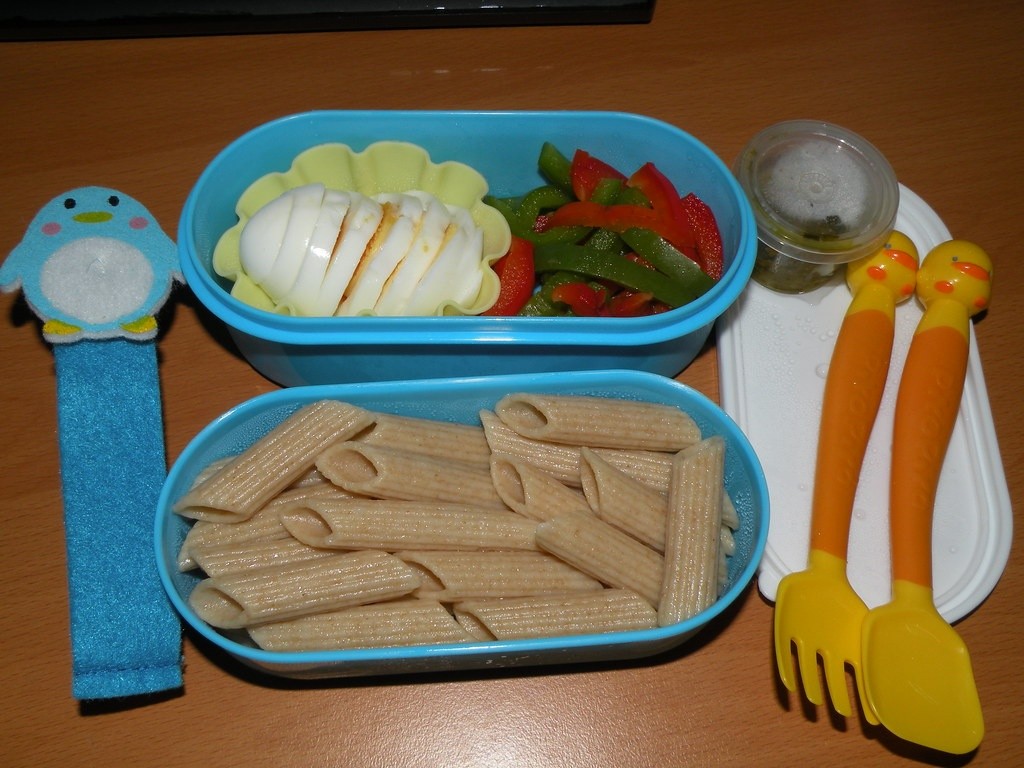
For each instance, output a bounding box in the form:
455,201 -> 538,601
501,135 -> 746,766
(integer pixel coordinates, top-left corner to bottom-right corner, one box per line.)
179,109 -> 758,389
152,367 -> 770,680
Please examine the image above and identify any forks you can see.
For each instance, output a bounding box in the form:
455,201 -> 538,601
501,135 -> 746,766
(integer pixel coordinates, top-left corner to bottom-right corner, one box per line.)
774,229 -> 920,729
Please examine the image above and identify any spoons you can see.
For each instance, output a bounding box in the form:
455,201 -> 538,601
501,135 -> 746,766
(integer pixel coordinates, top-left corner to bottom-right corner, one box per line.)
861,238 -> 994,755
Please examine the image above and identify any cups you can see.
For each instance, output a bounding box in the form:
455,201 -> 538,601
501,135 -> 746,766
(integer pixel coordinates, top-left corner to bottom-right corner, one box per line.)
737,121 -> 900,295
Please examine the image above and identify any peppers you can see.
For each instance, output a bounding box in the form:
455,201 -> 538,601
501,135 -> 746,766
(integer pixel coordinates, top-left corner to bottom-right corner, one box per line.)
478,142 -> 726,319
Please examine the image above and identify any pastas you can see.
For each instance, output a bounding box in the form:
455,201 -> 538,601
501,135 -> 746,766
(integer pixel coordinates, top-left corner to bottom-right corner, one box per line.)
173,393 -> 738,653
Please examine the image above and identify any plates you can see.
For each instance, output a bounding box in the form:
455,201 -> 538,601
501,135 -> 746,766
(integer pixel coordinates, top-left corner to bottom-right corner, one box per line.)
717,180 -> 1012,629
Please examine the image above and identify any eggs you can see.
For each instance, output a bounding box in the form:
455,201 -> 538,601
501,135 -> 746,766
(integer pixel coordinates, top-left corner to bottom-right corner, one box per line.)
238,182 -> 484,318
